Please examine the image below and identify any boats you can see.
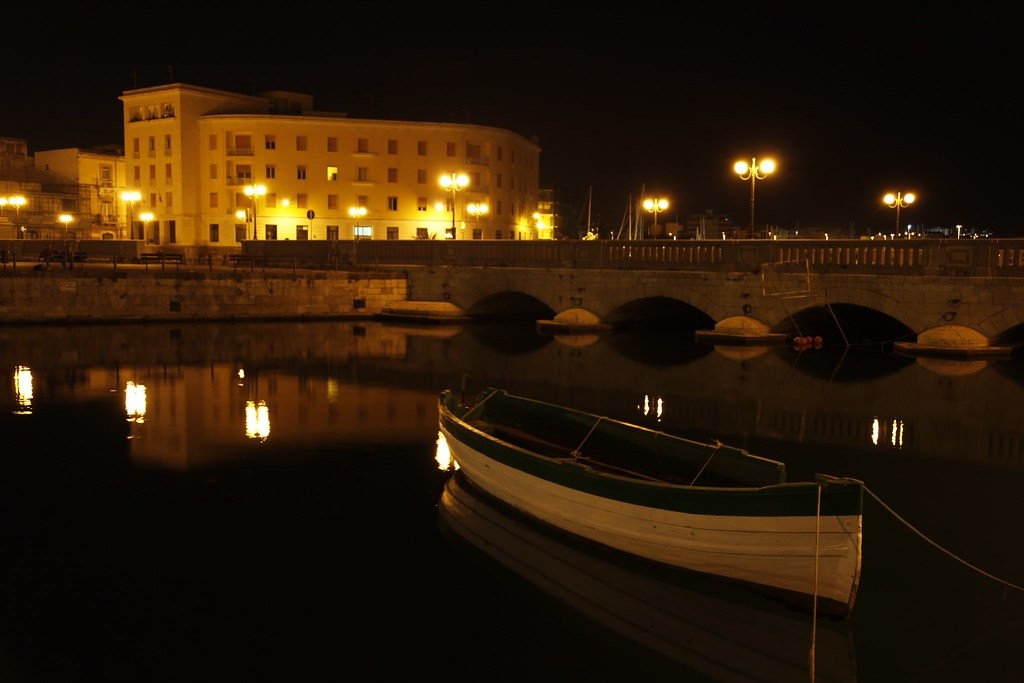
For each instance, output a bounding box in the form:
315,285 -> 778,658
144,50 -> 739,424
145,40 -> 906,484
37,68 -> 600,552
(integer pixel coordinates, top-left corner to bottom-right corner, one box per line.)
442,464 -> 857,683
436,381 -> 863,610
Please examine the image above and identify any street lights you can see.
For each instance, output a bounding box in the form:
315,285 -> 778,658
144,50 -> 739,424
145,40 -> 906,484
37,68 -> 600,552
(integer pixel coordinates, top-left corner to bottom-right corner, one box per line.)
642,196 -> 669,240
735,158 -> 774,236
140,212 -> 153,244
467,203 -> 489,231
121,187 -> 142,241
0,198 -> 8,216
60,215 -> 72,241
245,183 -> 266,239
884,190 -> 916,239
350,205 -> 366,239
10,197 -> 26,240
236,207 -> 252,241
437,170 -> 471,236
